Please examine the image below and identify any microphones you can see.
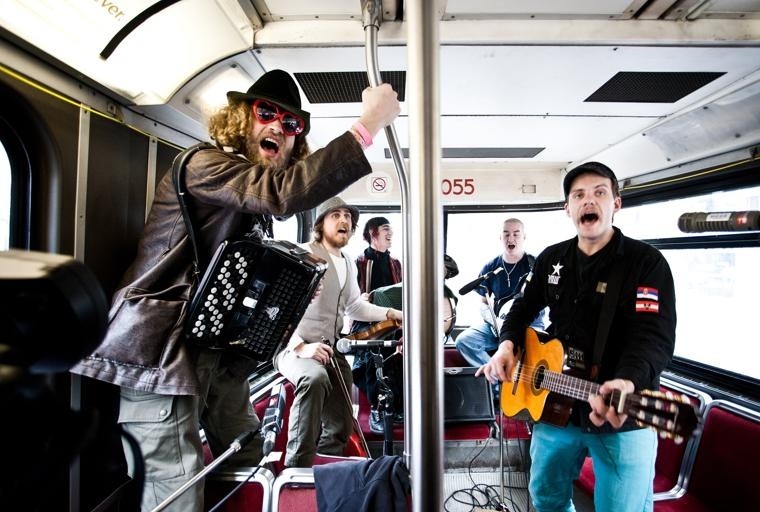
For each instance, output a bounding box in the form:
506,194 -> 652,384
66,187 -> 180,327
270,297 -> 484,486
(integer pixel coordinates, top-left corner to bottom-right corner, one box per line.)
460,271 -> 489,295
261,383 -> 286,456
337,337 -> 396,355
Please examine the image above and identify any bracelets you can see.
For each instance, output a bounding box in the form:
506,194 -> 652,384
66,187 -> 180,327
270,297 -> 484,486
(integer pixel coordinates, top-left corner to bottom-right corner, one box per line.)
349,119 -> 374,151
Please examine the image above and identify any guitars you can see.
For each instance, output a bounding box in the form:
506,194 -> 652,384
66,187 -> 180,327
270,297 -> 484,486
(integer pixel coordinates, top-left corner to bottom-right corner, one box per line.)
500,326 -> 702,444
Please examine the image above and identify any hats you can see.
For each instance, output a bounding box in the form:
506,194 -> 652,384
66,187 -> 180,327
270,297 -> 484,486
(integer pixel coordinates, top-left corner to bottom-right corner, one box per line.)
564,162 -> 616,195
313,197 -> 359,230
227,69 -> 310,136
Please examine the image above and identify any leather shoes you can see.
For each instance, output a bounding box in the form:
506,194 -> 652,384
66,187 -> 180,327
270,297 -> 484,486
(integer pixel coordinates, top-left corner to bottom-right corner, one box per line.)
381,412 -> 404,423
368,410 -> 384,434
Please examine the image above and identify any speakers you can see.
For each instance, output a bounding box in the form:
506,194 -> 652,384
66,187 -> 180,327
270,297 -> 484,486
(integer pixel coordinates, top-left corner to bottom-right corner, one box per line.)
445,367 -> 496,426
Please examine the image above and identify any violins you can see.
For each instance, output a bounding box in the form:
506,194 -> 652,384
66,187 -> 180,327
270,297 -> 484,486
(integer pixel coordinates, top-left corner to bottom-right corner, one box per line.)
341,319 -> 400,340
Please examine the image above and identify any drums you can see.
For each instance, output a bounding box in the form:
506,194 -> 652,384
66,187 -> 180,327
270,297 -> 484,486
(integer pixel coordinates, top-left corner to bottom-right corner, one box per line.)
443,285 -> 457,336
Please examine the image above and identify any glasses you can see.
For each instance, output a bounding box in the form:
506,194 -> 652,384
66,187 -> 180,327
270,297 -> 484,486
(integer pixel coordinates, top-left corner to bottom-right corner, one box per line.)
250,99 -> 304,136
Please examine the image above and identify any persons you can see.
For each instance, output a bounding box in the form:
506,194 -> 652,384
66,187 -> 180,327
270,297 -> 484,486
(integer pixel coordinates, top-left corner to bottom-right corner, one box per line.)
474,161 -> 677,512
350,217 -> 404,434
454,219 -> 546,392
68,67 -> 401,512
271,198 -> 403,488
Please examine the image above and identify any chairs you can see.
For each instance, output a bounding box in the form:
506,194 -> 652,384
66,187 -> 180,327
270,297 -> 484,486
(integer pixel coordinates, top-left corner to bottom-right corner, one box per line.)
199,347 -> 533,512
578,376 -> 760,512
